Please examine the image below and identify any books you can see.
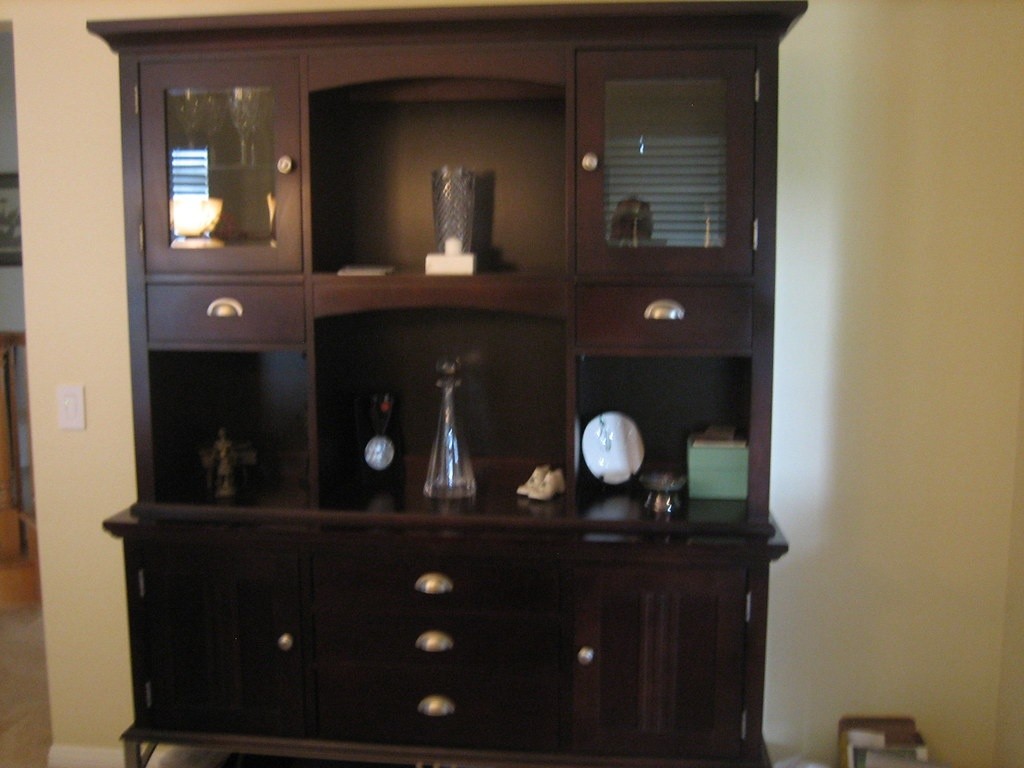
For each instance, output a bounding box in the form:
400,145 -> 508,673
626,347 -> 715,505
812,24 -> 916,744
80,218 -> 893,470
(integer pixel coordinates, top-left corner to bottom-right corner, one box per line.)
694,424 -> 746,448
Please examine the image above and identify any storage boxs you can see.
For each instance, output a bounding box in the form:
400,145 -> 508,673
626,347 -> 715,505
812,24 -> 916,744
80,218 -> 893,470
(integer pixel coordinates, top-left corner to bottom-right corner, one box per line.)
837,716 -> 928,768
686,435 -> 748,500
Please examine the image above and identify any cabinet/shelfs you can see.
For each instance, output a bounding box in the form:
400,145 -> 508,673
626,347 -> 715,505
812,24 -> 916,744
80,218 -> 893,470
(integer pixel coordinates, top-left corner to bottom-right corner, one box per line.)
87,0 -> 808,768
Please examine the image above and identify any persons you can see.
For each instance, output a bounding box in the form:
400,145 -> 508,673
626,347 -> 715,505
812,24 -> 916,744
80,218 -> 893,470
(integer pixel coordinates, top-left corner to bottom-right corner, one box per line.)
209,429 -> 238,491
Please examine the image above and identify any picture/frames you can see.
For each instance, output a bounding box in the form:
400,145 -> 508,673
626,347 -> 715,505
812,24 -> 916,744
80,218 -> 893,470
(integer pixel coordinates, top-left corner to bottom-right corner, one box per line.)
0,173 -> 22,265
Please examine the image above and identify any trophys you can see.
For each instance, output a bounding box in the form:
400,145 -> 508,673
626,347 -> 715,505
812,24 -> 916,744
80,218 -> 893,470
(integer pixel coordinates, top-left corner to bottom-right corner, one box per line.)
425,162 -> 478,275
424,358 -> 477,506
